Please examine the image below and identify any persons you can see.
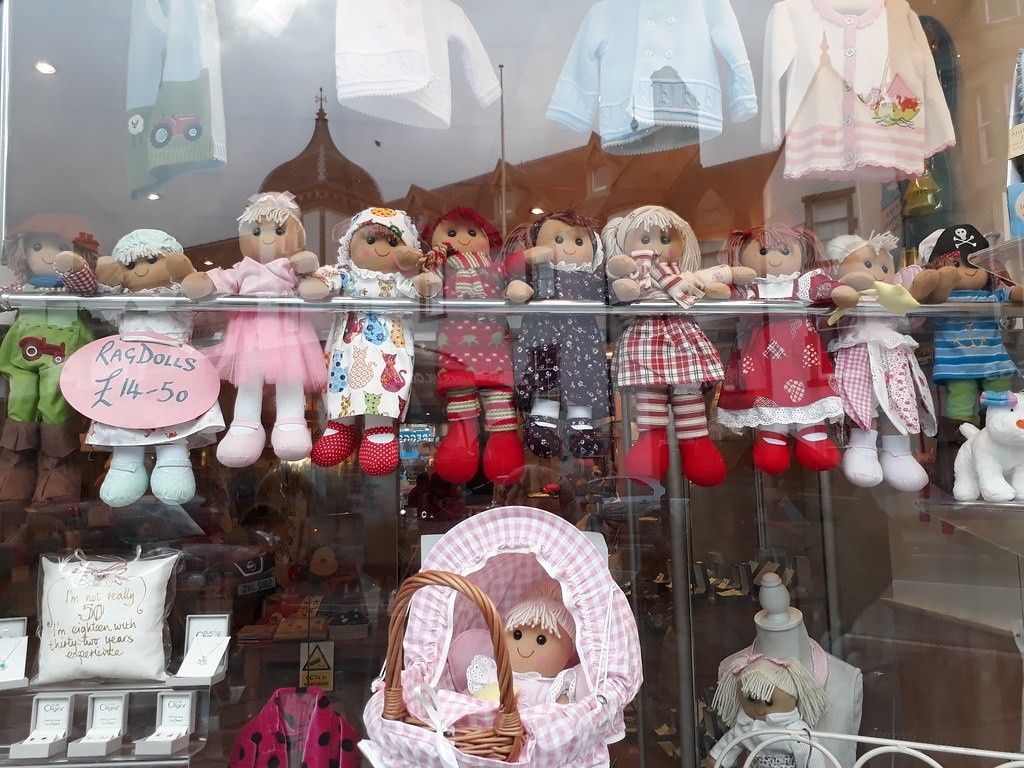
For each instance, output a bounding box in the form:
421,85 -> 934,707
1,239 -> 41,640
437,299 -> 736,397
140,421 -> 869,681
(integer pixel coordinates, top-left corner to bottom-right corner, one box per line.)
0,191 -> 1024,506
706,653 -> 828,768
459,579 -> 576,709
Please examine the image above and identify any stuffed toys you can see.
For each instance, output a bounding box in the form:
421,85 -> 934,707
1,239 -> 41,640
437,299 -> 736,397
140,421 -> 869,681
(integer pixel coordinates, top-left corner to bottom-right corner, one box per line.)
300,542 -> 363,625
953,391 -> 1024,503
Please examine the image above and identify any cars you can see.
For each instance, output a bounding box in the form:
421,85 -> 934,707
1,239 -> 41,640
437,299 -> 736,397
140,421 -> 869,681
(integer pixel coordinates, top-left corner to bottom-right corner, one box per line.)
574,472 -> 668,525
22,495 -> 290,637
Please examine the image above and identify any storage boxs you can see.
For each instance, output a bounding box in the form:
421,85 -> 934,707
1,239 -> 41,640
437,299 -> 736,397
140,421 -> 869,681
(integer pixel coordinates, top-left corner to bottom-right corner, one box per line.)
9,692 -> 74,759
166,613 -> 231,686
134,689 -> 198,755
0,616 -> 30,692
66,691 -> 131,757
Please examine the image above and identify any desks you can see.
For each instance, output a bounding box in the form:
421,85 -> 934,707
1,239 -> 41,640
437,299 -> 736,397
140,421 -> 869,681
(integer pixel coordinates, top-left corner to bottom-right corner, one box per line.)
239,618 -> 391,698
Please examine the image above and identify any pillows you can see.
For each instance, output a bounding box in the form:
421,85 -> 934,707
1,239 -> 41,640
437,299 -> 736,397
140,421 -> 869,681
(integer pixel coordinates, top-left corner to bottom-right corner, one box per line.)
32,554 -> 178,686
